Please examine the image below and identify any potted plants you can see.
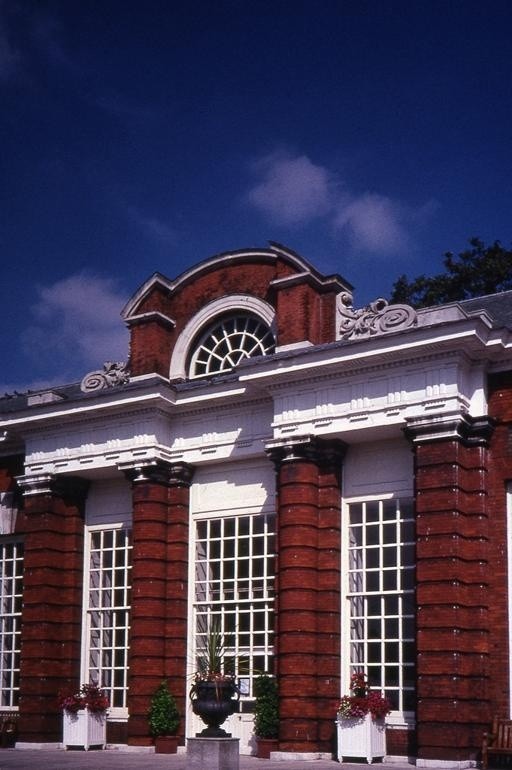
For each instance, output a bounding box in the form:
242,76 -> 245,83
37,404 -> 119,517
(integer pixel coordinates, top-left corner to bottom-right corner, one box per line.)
142,676 -> 182,754
253,672 -> 281,759
189,624 -> 250,738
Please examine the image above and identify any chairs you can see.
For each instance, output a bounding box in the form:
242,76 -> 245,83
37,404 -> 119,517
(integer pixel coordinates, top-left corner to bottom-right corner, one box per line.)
481,715 -> 512,770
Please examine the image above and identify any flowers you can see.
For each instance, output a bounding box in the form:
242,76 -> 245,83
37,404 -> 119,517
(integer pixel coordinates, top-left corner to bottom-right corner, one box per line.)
62,682 -> 110,713
334,673 -> 392,719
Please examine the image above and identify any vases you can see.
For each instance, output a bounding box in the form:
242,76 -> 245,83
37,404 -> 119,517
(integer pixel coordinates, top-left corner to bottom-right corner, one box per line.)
63,708 -> 107,752
334,712 -> 387,765
